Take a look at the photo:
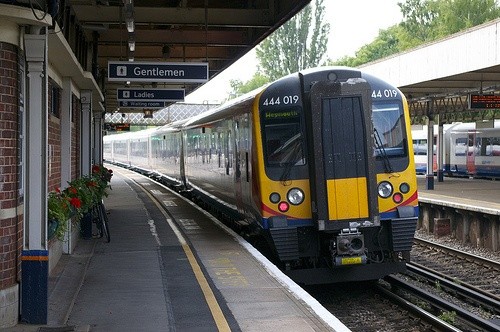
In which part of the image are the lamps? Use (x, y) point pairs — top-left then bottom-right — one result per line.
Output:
(125, 1), (136, 62)
(143, 110), (154, 118)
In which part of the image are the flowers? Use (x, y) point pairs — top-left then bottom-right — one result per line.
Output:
(48, 165), (113, 240)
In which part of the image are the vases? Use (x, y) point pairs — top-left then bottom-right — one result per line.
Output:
(48, 221), (58, 240)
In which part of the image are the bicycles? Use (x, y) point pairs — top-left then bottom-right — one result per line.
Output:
(82, 172), (112, 242)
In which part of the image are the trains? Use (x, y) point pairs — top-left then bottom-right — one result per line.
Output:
(409, 118), (500, 178)
(102, 66), (419, 286)
